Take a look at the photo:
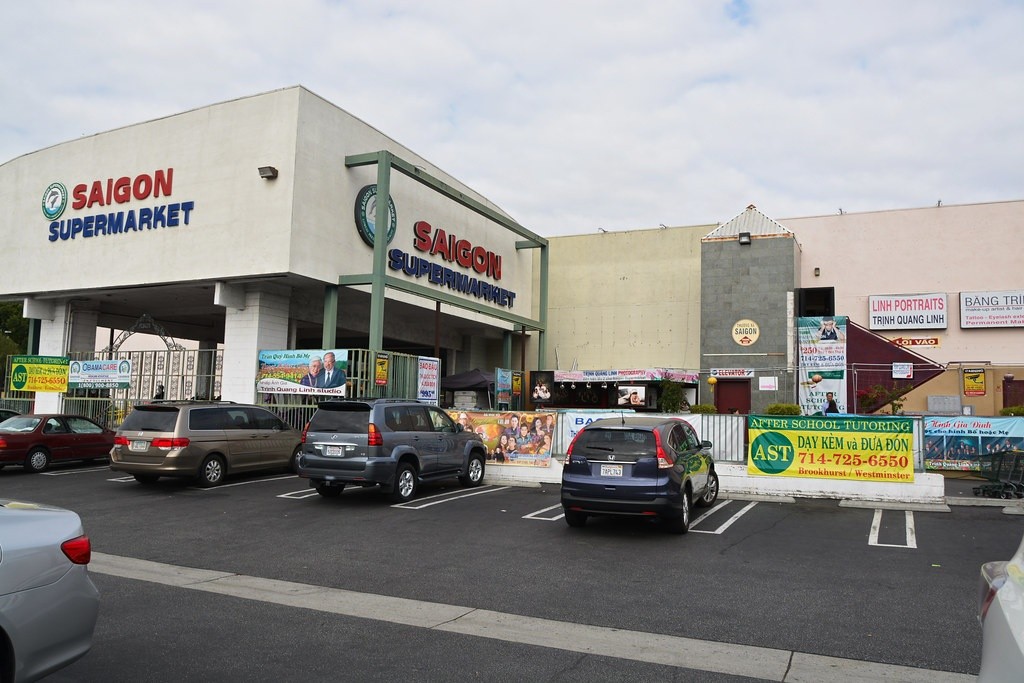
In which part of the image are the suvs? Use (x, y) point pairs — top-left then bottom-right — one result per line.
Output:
(299, 396), (488, 504)
(108, 398), (304, 488)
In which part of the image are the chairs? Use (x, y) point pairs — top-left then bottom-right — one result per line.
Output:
(234, 416), (246, 427)
(45, 423), (52, 431)
(408, 416), (418, 425)
(611, 434), (625, 442)
(60, 424), (69, 429)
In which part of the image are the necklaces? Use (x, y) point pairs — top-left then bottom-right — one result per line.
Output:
(307, 371), (318, 388)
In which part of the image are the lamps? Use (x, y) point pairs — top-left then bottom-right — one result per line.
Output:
(738, 232), (751, 245)
(601, 382), (607, 389)
(586, 382), (591, 389)
(659, 224), (666, 228)
(815, 268), (820, 276)
(599, 228), (607, 232)
(838, 209), (843, 215)
(560, 382), (565, 389)
(614, 382), (619, 387)
(257, 166), (277, 179)
(937, 201), (942, 206)
(571, 382), (576, 389)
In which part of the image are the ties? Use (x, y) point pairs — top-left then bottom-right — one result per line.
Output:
(326, 372), (331, 386)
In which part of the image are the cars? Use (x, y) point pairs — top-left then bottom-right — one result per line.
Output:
(561, 416), (719, 535)
(0, 497), (101, 683)
(0, 414), (117, 472)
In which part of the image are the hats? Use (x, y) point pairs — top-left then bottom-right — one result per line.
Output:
(630, 389), (638, 394)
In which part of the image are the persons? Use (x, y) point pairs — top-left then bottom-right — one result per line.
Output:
(152, 385), (165, 400)
(299, 352), (345, 391)
(627, 391), (642, 406)
(925, 438), (1020, 462)
(728, 408), (740, 414)
(816, 317), (845, 343)
(819, 392), (840, 415)
(457, 412), (555, 465)
(533, 383), (551, 399)
